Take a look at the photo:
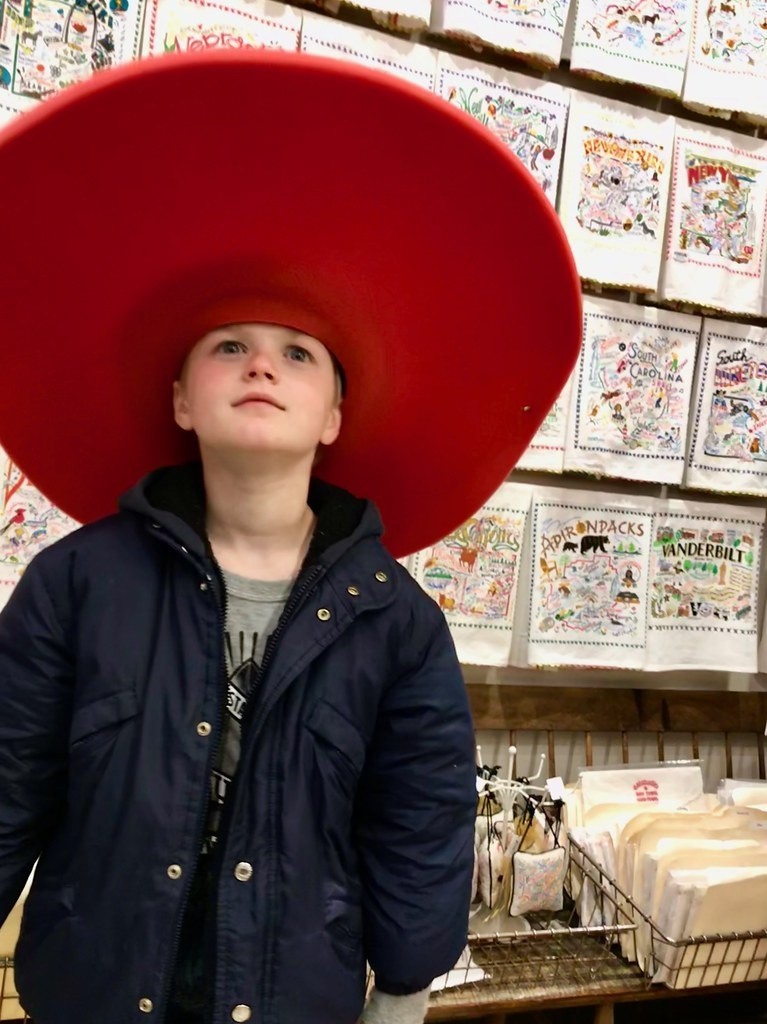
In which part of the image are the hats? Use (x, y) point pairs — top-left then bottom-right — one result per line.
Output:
(2, 48), (582, 558)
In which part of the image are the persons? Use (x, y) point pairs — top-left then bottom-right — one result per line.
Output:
(0, 48), (583, 1024)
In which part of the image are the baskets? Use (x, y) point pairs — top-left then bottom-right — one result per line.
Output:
(567, 828), (767, 989)
(365, 805), (650, 1009)
